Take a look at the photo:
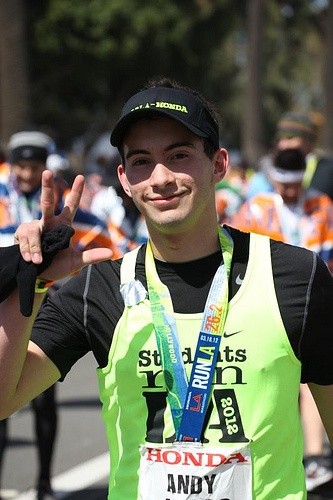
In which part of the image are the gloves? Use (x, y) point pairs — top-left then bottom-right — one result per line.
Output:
(0, 224), (76, 316)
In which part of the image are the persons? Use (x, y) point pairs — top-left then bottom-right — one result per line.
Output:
(231, 148), (333, 492)
(46, 108), (333, 262)
(1, 82), (333, 500)
(0, 130), (78, 500)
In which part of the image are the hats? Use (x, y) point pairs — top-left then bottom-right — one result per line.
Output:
(110, 87), (220, 152)
(276, 112), (318, 142)
(8, 130), (58, 166)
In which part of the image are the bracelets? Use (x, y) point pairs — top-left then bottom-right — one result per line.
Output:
(34, 278), (55, 295)
